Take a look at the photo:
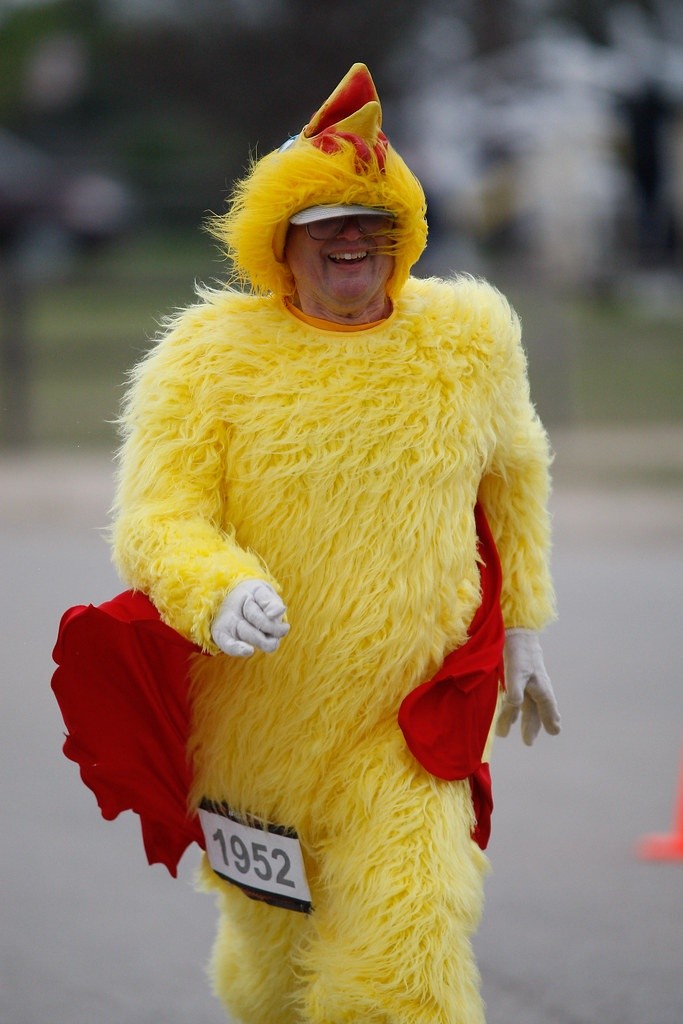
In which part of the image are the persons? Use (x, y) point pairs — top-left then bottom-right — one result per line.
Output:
(50, 56), (559, 1024)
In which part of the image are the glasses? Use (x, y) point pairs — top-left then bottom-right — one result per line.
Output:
(307, 214), (394, 238)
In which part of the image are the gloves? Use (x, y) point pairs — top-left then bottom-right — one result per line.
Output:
(213, 577), (289, 659)
(499, 629), (560, 748)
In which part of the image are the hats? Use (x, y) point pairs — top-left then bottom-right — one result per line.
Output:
(288, 201), (396, 224)
